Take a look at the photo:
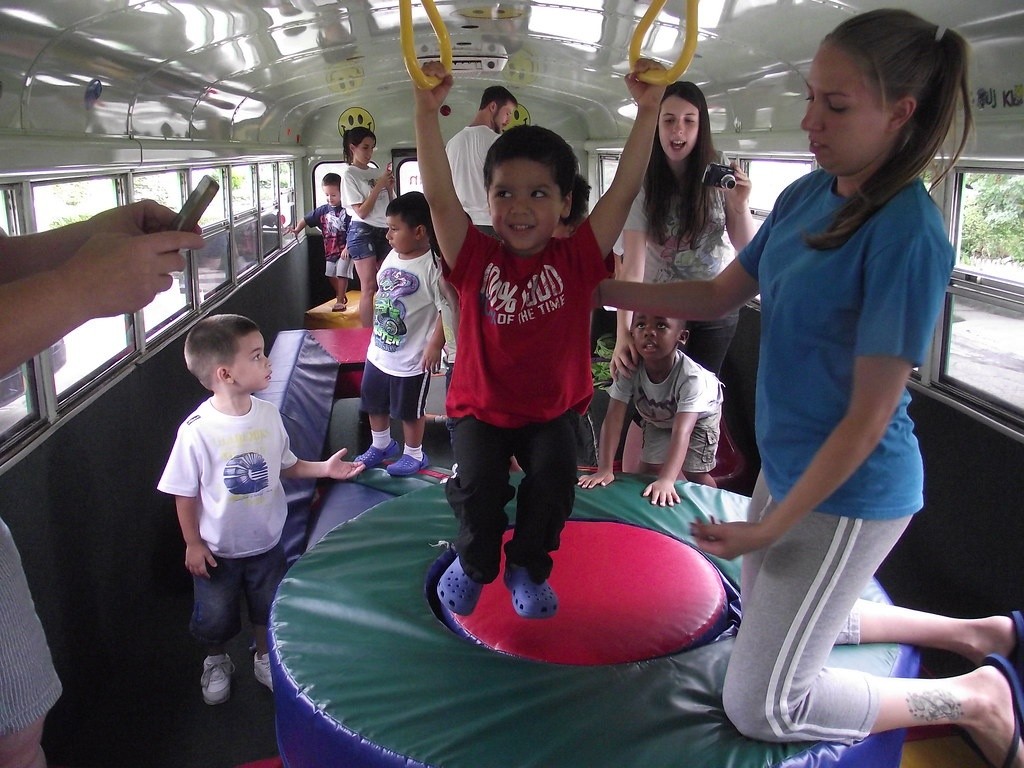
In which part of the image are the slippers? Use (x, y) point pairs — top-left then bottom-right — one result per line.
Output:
(961, 653), (1024, 768)
(1009, 610), (1024, 679)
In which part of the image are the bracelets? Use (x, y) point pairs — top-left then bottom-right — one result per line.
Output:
(598, 283), (604, 306)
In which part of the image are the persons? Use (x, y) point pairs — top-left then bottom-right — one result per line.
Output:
(552, 174), (591, 239)
(288, 173), (354, 312)
(595, 8), (1024, 768)
(157, 313), (366, 705)
(445, 85), (518, 240)
(0, 199), (207, 768)
(356, 190), (446, 476)
(610, 81), (756, 474)
(576, 310), (723, 507)
(341, 127), (397, 328)
(412, 58), (668, 619)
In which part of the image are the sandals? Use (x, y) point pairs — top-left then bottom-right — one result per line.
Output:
(436, 555), (481, 615)
(387, 452), (429, 476)
(354, 438), (400, 468)
(504, 561), (558, 619)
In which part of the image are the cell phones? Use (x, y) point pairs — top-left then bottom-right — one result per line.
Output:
(166, 174), (220, 254)
(387, 162), (392, 173)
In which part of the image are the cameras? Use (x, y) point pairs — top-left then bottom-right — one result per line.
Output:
(700, 162), (738, 191)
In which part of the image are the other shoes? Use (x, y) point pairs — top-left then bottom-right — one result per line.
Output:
(344, 297), (348, 304)
(332, 303), (346, 312)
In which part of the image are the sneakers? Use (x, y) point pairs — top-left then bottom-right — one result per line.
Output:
(253, 652), (274, 691)
(201, 653), (236, 705)
